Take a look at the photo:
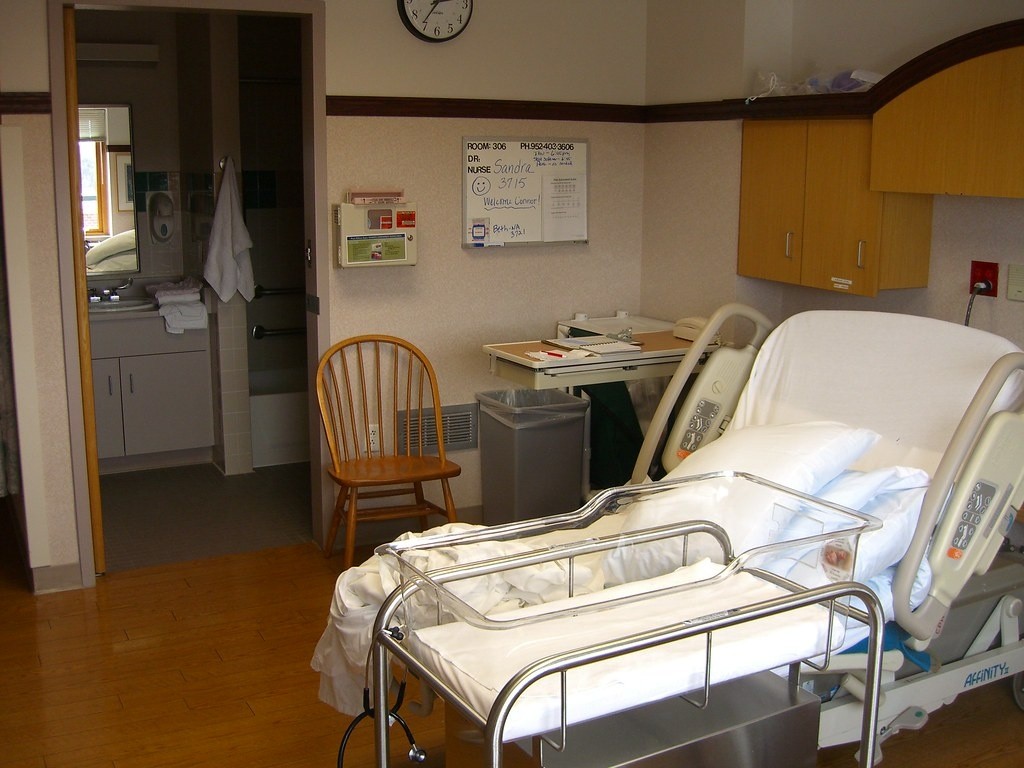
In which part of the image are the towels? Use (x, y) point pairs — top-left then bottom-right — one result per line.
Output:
(147, 276), (209, 335)
(202, 156), (258, 303)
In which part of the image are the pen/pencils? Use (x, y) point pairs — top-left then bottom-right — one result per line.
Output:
(540, 349), (566, 358)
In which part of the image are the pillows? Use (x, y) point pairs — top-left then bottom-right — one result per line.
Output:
(599, 420), (882, 588)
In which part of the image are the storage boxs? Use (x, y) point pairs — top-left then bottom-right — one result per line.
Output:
(331, 202), (418, 267)
(373, 466), (883, 739)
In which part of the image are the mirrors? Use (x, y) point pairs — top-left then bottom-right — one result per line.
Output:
(78, 103), (142, 275)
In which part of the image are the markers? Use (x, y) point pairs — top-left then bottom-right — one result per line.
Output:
(474, 243), (505, 247)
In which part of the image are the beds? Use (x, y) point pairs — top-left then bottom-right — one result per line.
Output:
(308, 306), (1024, 768)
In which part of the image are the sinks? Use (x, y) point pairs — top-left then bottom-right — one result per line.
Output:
(86, 297), (156, 312)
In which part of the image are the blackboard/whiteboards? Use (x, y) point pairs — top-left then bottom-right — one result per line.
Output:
(461, 137), (590, 249)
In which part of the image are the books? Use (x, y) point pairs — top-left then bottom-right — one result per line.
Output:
(543, 334), (641, 356)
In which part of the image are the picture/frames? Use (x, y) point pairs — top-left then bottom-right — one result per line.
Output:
(115, 153), (134, 213)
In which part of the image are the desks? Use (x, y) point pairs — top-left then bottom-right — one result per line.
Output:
(482, 330), (733, 497)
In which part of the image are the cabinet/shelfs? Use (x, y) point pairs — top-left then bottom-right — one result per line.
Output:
(738, 116), (931, 298)
(90, 315), (216, 476)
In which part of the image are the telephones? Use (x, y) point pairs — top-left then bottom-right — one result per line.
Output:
(672, 316), (724, 346)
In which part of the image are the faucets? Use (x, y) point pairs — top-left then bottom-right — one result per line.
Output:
(103, 276), (133, 302)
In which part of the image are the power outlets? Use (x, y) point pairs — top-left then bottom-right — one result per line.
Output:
(363, 424), (380, 452)
(967, 260), (999, 297)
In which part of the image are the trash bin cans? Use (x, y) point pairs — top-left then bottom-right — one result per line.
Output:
(474, 389), (588, 527)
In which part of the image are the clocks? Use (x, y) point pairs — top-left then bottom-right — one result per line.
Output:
(397, 0), (473, 43)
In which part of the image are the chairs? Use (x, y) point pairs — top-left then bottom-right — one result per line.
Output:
(315, 334), (463, 570)
